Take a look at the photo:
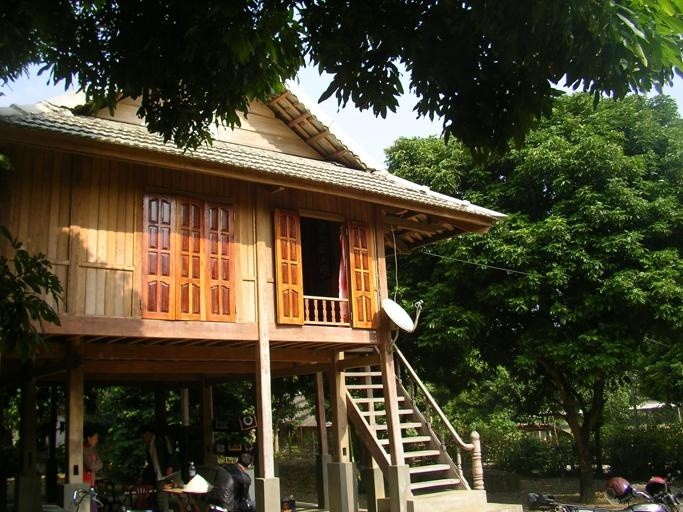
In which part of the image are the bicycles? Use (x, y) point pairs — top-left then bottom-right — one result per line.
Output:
(38, 487), (155, 512)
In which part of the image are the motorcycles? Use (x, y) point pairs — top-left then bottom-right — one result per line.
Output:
(522, 478), (682, 512)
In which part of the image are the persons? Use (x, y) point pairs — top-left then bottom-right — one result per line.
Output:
(135, 427), (175, 486)
(82, 426), (103, 512)
(207, 452), (252, 512)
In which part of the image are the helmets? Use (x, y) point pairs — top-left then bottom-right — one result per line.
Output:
(605, 477), (633, 506)
